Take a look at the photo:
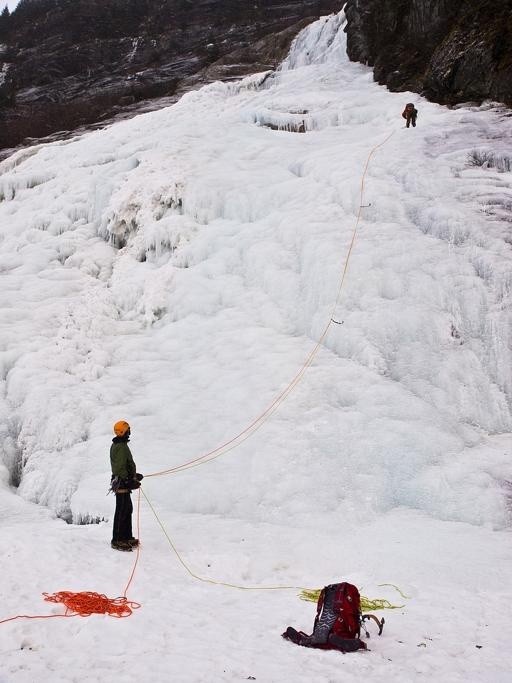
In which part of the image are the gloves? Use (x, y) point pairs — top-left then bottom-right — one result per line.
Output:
(134, 473), (143, 488)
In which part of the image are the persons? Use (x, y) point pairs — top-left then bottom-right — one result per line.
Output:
(111, 421), (139, 552)
(402, 103), (418, 128)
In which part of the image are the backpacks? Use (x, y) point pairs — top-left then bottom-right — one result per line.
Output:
(312, 582), (362, 653)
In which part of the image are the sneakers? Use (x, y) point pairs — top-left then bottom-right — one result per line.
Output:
(111, 537), (138, 550)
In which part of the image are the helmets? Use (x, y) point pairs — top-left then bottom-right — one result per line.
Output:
(114, 421), (129, 436)
